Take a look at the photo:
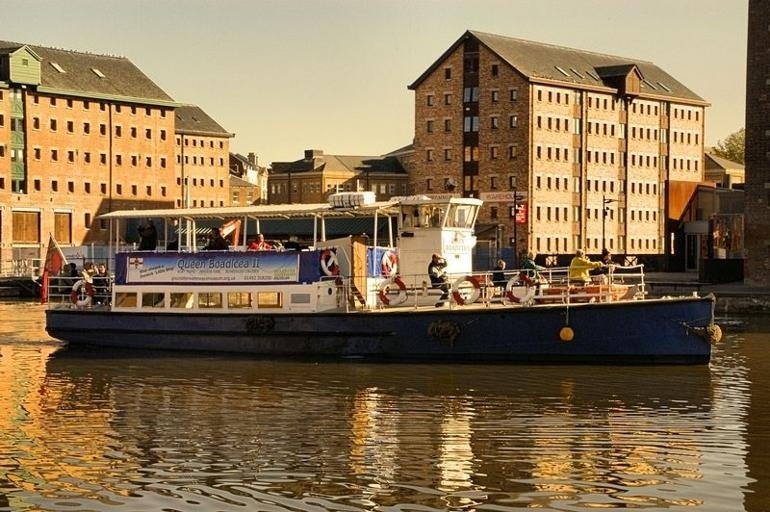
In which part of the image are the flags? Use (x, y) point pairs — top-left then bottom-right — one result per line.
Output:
(41, 237), (63, 305)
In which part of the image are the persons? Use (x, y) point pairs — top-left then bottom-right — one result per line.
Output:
(492, 259), (510, 296)
(201, 227), (229, 251)
(521, 249), (547, 297)
(137, 219), (158, 250)
(284, 236), (302, 250)
(569, 248), (605, 286)
(167, 233), (183, 251)
(595, 250), (622, 284)
(248, 233), (272, 251)
(271, 239), (286, 251)
(428, 254), (450, 307)
(57, 261), (113, 306)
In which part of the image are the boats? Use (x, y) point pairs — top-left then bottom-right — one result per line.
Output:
(46, 191), (716, 366)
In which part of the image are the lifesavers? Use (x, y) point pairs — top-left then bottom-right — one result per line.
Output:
(506, 274), (536, 303)
(380, 279), (406, 306)
(321, 250), (339, 276)
(382, 251), (397, 278)
(71, 280), (93, 306)
(452, 276), (480, 305)
